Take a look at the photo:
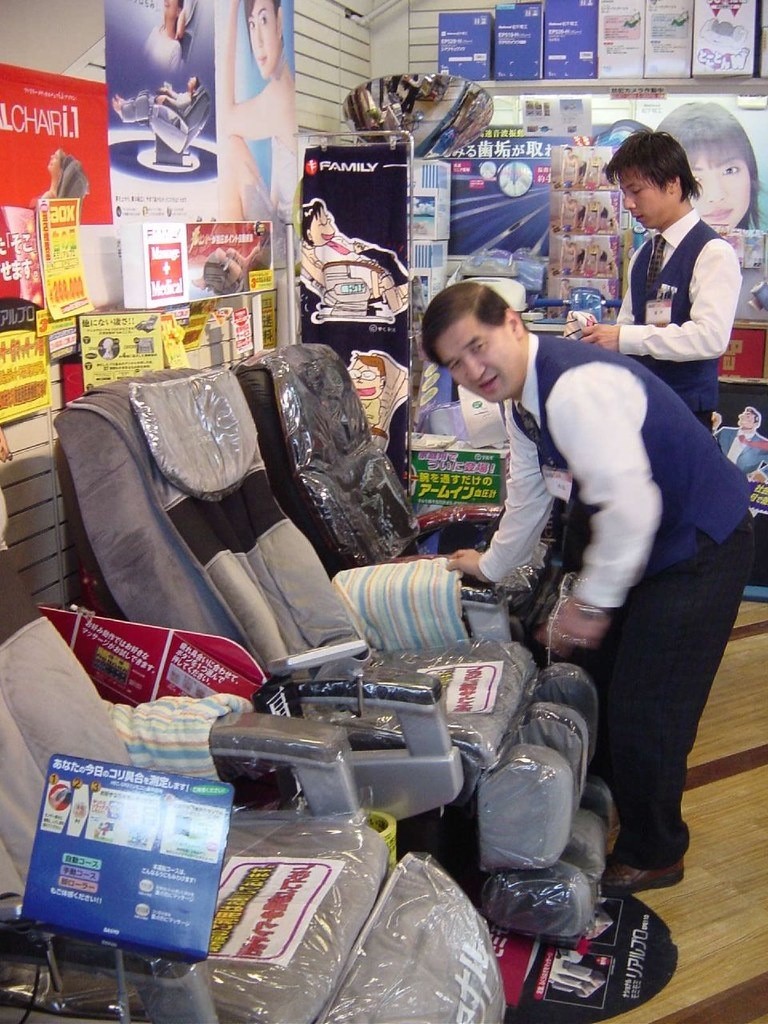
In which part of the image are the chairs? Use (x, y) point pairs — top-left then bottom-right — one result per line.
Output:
(51, 367), (618, 938)
(236, 344), (567, 632)
(0, 492), (505, 1024)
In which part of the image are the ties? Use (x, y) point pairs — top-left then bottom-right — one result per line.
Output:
(517, 400), (544, 451)
(645, 235), (666, 295)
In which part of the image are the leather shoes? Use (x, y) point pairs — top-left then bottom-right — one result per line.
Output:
(599, 853), (685, 900)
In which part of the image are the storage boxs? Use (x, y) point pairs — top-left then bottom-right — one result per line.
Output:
(494, 2), (543, 81)
(166, 297), (254, 369)
(437, 12), (494, 80)
(544, 0), (768, 80)
(121, 218), (274, 309)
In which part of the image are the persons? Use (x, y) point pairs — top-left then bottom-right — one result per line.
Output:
(421, 282), (756, 894)
(217, 0), (298, 266)
(41, 148), (67, 199)
(655, 101), (760, 229)
(112, 76), (200, 119)
(581, 130), (743, 431)
(145, 0), (183, 73)
